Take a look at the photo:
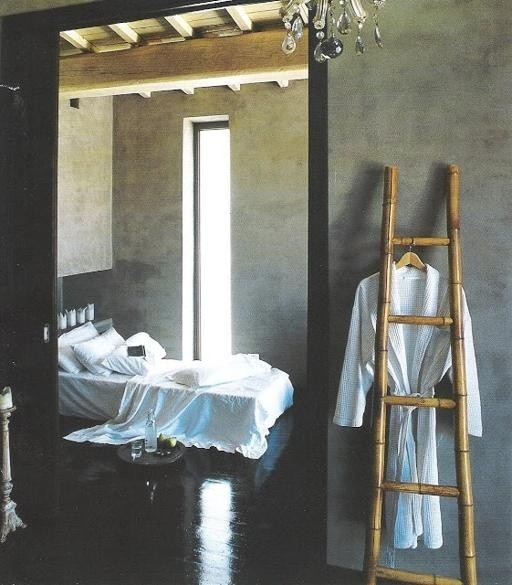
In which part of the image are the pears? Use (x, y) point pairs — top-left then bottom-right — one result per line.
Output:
(166, 438), (177, 447)
(160, 434), (168, 445)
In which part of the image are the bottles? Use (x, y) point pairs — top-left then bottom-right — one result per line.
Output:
(144, 409), (157, 453)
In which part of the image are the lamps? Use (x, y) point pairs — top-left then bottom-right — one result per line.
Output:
(279, 0), (388, 63)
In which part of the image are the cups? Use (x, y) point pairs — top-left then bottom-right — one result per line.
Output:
(130, 440), (142, 458)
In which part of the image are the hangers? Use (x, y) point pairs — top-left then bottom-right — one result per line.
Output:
(395, 238), (428, 273)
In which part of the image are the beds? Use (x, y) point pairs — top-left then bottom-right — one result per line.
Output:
(58, 360), (296, 460)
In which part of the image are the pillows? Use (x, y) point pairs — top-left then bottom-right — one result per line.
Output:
(161, 353), (271, 388)
(71, 327), (126, 376)
(58, 321), (100, 374)
(102, 332), (166, 376)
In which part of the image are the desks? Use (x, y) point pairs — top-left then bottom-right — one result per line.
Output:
(115, 437), (198, 500)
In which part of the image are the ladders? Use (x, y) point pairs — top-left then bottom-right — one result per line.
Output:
(365, 163), (478, 585)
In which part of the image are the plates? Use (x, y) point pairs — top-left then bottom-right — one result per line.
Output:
(117, 440), (186, 465)
